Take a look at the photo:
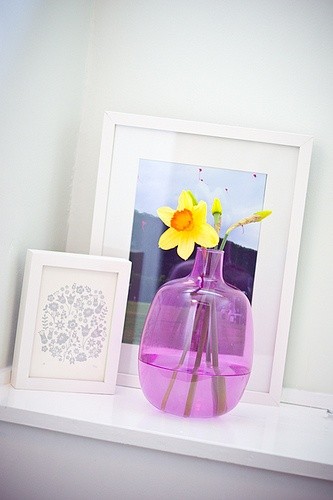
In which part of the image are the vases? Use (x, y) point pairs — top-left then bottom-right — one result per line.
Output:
(138, 247), (254, 419)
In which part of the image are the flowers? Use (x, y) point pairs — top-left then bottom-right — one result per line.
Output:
(156, 190), (273, 418)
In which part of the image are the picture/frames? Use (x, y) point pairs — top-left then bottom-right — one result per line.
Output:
(88, 111), (313, 408)
(9, 249), (132, 394)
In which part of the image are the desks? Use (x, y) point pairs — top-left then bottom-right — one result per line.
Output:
(0, 365), (333, 500)
